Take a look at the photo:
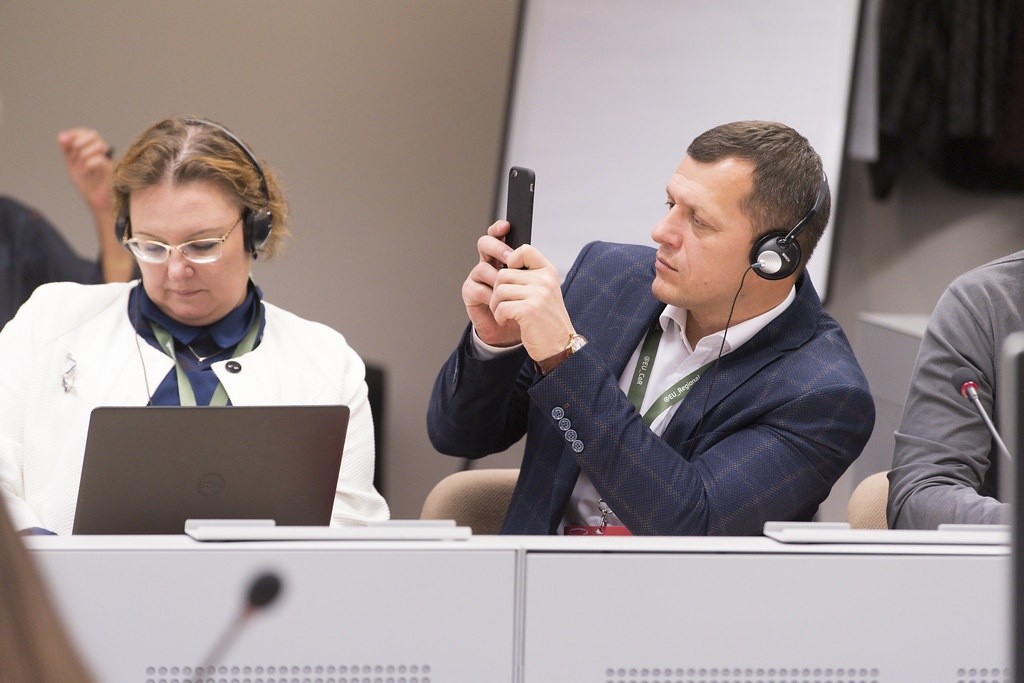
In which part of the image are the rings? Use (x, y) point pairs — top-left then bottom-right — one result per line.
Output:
(106, 146), (114, 157)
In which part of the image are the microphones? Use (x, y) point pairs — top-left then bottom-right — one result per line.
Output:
(951, 367), (1013, 465)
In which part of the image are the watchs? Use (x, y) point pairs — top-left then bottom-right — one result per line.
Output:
(537, 334), (589, 376)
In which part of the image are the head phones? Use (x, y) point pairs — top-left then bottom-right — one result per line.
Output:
(749, 172), (828, 281)
(113, 120), (273, 261)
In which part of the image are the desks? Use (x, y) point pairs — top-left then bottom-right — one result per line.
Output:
(854, 311), (938, 497)
(14, 534), (1013, 683)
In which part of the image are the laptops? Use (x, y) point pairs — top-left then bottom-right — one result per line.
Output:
(71, 405), (350, 534)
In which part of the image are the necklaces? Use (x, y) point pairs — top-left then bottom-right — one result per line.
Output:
(187, 345), (223, 364)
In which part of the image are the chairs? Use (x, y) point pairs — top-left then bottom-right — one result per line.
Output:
(849, 471), (889, 527)
(419, 464), (518, 528)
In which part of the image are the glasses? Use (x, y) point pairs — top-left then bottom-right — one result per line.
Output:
(120, 208), (246, 265)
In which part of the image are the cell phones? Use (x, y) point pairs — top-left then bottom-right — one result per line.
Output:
(503, 167), (536, 270)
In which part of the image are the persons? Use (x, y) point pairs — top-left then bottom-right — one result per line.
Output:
(0, 119), (390, 535)
(886, 250), (1024, 531)
(0, 128), (142, 333)
(426, 120), (875, 538)
